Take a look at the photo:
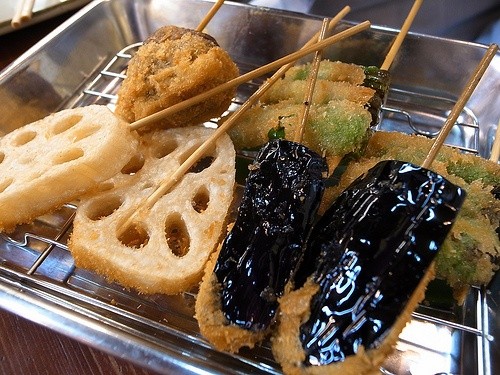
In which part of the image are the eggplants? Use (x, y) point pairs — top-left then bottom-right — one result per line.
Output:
(267, 160), (466, 375)
(197, 138), (329, 353)
